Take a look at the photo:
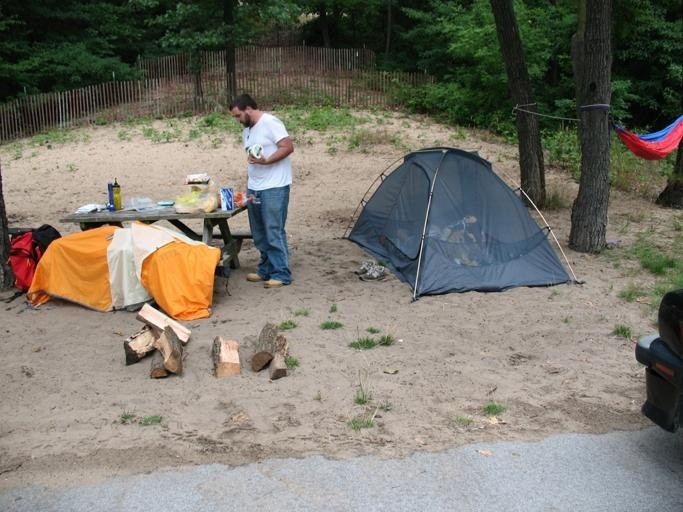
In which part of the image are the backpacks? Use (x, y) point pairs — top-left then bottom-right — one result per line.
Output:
(10, 224), (62, 290)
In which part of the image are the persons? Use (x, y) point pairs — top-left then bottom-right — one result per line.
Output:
(230, 93), (294, 287)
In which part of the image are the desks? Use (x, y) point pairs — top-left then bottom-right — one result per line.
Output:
(59, 203), (253, 278)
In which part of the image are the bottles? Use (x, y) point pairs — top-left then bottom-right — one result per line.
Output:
(233, 195), (253, 209)
(111, 178), (121, 211)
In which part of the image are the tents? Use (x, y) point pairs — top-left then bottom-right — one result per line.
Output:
(348, 147), (572, 295)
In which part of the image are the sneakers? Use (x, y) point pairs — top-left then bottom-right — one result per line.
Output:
(356, 260), (384, 282)
(264, 279), (283, 288)
(247, 273), (265, 282)
